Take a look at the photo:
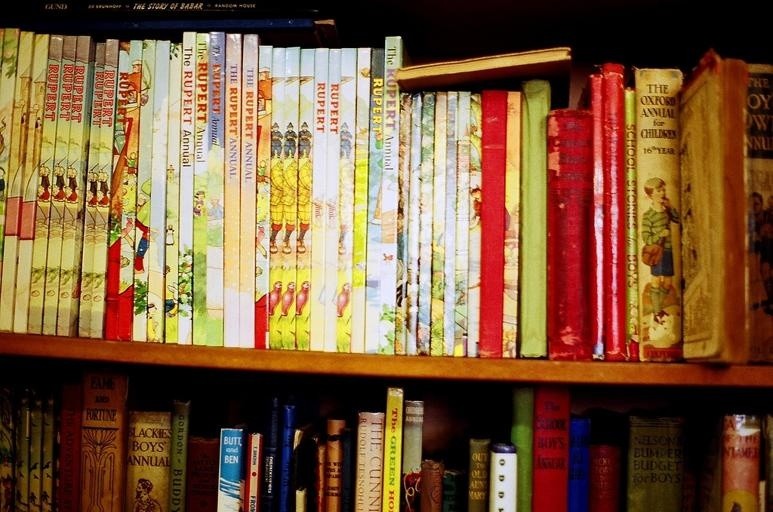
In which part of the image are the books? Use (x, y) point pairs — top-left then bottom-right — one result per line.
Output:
(2, 29), (772, 511)
